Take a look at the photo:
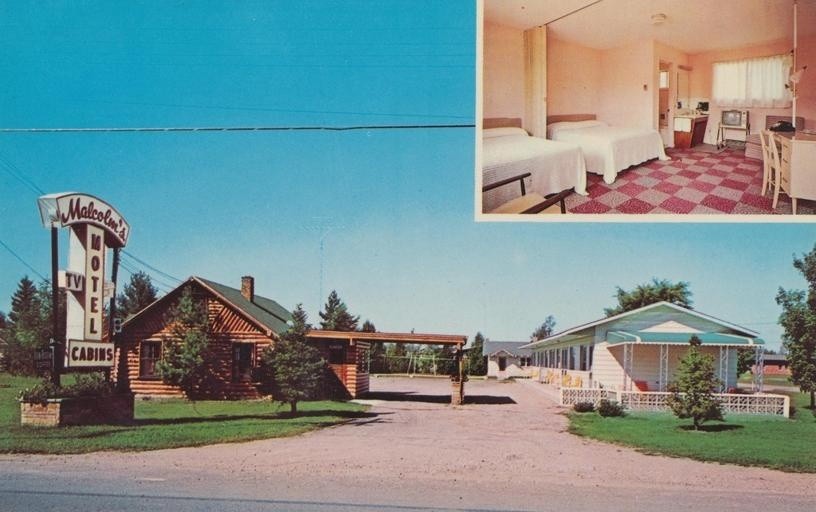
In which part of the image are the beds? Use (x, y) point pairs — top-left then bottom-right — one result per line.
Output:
(546, 115), (672, 185)
(482, 117), (582, 214)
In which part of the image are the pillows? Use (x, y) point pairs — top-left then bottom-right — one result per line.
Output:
(482, 126), (528, 139)
(548, 120), (609, 131)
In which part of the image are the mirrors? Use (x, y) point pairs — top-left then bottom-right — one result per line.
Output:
(676, 72), (691, 108)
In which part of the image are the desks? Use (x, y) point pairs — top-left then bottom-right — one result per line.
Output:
(674, 113), (708, 148)
(761, 126), (815, 215)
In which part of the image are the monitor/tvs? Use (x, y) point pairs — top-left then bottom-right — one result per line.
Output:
(722, 111), (749, 128)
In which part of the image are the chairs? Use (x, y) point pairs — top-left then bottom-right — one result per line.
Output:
(482, 172), (574, 214)
(752, 130), (783, 208)
(744, 116), (804, 163)
